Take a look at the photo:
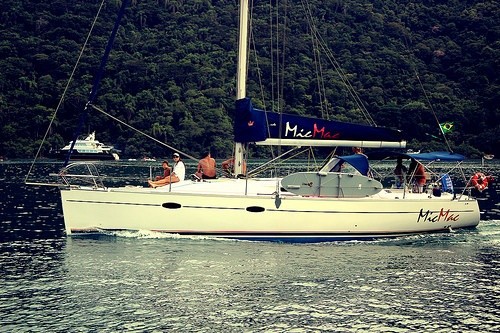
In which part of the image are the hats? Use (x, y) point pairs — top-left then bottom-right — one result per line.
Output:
(173, 152), (180, 156)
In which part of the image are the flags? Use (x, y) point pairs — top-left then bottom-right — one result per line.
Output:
(439, 121), (456, 134)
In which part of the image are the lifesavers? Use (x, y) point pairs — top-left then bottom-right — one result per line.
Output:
(473, 172), (488, 191)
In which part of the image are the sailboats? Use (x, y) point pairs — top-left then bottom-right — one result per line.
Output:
(24, 0), (480, 236)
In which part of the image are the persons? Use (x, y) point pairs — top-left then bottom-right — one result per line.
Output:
(148, 153), (185, 188)
(195, 151), (216, 179)
(148, 161), (171, 188)
(406, 158), (426, 193)
(354, 148), (368, 175)
(394, 158), (407, 189)
(222, 153), (246, 178)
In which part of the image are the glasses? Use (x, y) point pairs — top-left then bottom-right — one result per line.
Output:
(173, 156), (179, 158)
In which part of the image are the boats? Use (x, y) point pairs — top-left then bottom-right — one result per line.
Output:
(60, 130), (120, 161)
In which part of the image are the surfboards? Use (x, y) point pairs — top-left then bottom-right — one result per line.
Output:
(281, 172), (383, 198)
